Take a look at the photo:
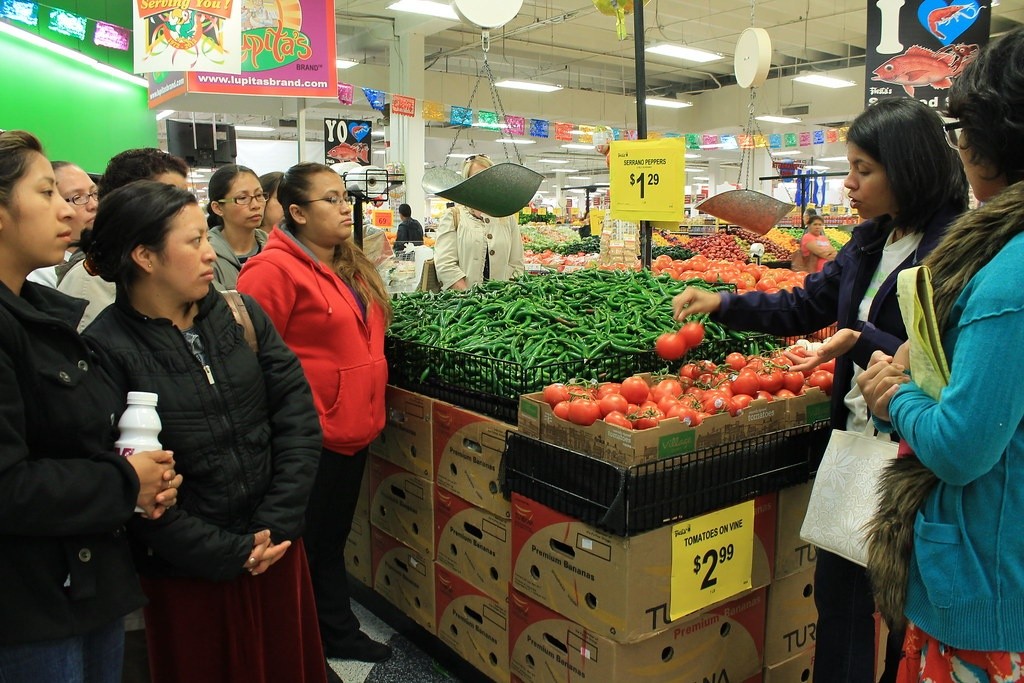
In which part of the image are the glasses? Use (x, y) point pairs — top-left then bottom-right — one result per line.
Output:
(297, 195), (357, 207)
(65, 189), (99, 205)
(218, 192), (270, 205)
(942, 119), (967, 151)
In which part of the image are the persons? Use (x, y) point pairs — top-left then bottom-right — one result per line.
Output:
(56, 147), (188, 335)
(803, 208), (825, 235)
(236, 161), (393, 683)
(579, 212), (591, 241)
(206, 164), (270, 292)
(26, 161), (98, 289)
(82, 179), (328, 683)
(856, 31), (1024, 683)
(800, 216), (838, 273)
(393, 204), (423, 251)
(258, 172), (285, 235)
(0, 129), (183, 683)
(670, 97), (969, 683)
(434, 152), (525, 292)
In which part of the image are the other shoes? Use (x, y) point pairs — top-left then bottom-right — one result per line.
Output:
(327, 665), (344, 683)
(324, 629), (391, 661)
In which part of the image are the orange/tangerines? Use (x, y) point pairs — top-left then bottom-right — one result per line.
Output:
(423, 237), (435, 246)
(386, 232), (397, 246)
(765, 229), (804, 252)
(824, 227), (851, 251)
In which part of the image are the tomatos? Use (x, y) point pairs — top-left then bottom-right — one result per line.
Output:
(596, 254), (812, 293)
(542, 321), (836, 434)
(524, 250), (596, 270)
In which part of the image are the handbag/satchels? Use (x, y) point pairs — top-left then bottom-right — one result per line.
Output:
(417, 209), (461, 291)
(800, 416), (900, 570)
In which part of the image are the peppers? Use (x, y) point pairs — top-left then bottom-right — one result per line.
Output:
(380, 263), (781, 402)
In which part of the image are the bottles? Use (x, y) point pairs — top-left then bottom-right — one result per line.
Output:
(115, 391), (163, 513)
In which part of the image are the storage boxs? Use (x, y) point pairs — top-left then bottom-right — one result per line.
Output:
(345, 385), (890, 683)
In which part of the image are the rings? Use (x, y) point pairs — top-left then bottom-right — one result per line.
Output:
(168, 480), (171, 487)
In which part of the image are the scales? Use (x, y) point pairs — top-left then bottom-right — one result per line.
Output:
(420, 0), (545, 218)
(694, 27), (797, 236)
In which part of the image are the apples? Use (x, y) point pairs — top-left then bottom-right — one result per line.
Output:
(682, 227), (794, 263)
(520, 226), (581, 254)
